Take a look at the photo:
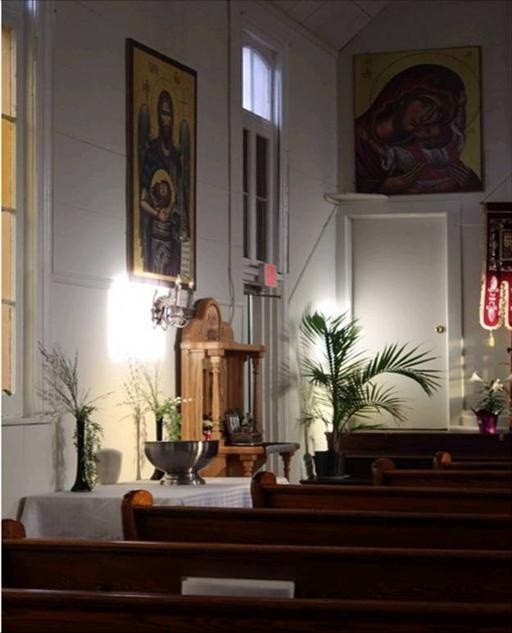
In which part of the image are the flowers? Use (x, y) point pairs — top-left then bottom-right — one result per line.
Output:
(468, 370), (508, 410)
(34, 340), (115, 493)
(139, 363), (187, 440)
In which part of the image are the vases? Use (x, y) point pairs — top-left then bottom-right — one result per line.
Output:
(149, 418), (165, 480)
(70, 420), (91, 492)
(476, 412), (500, 436)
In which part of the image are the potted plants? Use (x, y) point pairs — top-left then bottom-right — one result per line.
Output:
(287, 306), (444, 477)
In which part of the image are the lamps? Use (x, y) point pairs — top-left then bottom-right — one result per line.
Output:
(151, 274), (196, 331)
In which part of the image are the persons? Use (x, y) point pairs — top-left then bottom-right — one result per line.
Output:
(138, 86), (191, 278)
(355, 63), (481, 190)
(380, 88), (469, 174)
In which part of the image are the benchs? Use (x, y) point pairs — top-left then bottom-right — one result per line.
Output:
(1, 451), (512, 633)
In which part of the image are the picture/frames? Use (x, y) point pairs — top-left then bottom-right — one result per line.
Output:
(225, 413), (240, 435)
(125, 38), (198, 290)
